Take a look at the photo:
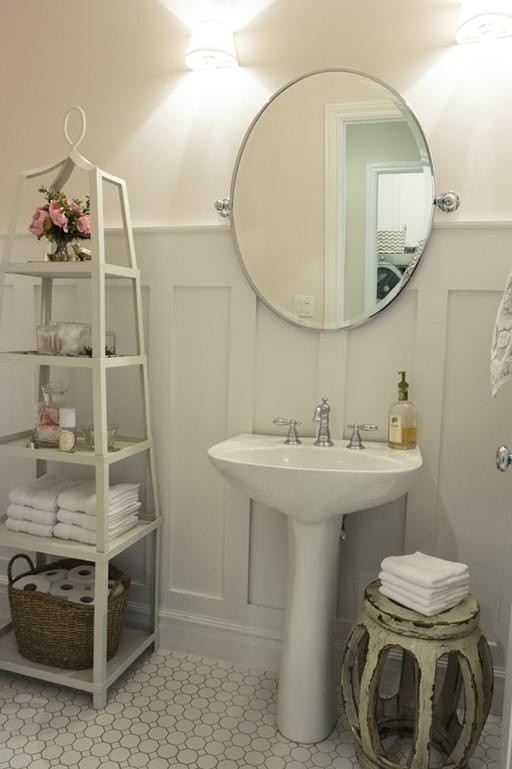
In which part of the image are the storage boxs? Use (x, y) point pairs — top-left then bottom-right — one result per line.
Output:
(373, 222), (409, 255)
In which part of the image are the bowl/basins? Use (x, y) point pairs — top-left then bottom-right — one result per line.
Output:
(83, 421), (121, 449)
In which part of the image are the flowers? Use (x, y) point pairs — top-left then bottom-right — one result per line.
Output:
(30, 183), (92, 246)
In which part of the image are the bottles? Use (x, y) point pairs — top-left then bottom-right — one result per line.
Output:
(30, 379), (70, 450)
(36, 321), (61, 356)
(59, 407), (77, 450)
(103, 333), (117, 354)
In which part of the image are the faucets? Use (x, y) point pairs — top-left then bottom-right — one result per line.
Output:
(313, 397), (333, 447)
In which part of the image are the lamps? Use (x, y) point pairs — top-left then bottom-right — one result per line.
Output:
(181, 18), (236, 77)
(451, 1), (512, 50)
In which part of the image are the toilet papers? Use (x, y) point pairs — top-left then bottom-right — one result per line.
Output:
(11, 565), (125, 606)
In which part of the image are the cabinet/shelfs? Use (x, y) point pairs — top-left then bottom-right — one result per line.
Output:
(0, 104), (167, 705)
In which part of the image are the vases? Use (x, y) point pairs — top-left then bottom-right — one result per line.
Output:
(51, 238), (69, 261)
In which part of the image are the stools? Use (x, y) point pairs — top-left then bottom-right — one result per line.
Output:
(338, 580), (497, 769)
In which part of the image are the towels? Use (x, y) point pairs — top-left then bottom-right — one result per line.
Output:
(490, 270), (511, 401)
(375, 549), (472, 619)
(4, 469), (143, 545)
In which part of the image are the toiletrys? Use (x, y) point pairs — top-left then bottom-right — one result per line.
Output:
(389, 371), (417, 451)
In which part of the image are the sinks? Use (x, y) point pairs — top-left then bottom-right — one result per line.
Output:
(208, 432), (423, 521)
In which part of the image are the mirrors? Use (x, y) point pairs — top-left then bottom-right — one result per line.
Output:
(215, 65), (462, 337)
